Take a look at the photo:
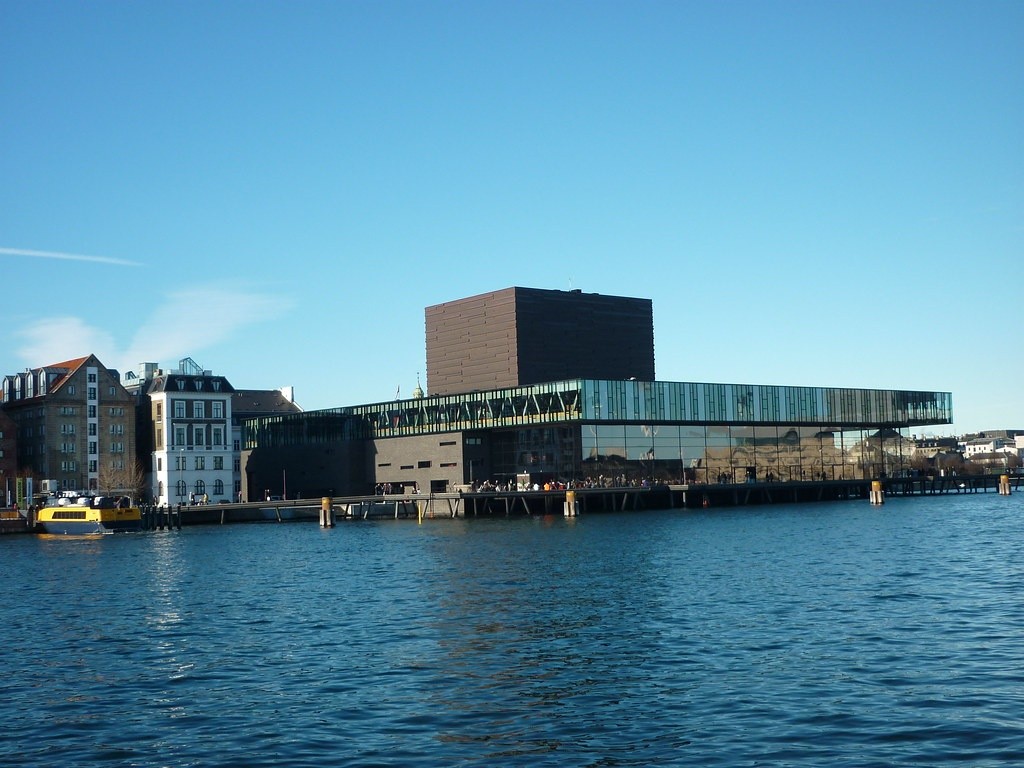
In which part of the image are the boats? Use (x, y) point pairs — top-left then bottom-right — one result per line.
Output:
(37, 495), (142, 535)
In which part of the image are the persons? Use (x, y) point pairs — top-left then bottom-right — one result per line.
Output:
(547, 473), (690, 487)
(374, 482), (393, 495)
(189, 492), (202, 506)
(717, 472), (731, 484)
(238, 491), (242, 503)
(821, 472), (827, 481)
(906, 468), (913, 478)
(945, 465), (957, 477)
(879, 470), (886, 478)
(203, 493), (208, 505)
(473, 479), (500, 492)
(766, 472), (774, 483)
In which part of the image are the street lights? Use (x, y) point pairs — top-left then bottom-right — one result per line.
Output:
(180, 448), (185, 507)
